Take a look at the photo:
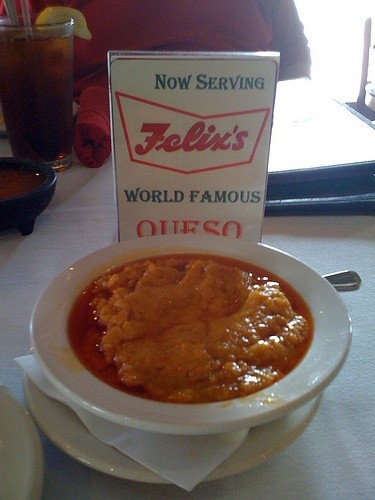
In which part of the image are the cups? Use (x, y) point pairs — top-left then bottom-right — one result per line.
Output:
(0, 13), (75, 171)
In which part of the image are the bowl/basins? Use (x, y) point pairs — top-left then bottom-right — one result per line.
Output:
(0, 156), (57, 236)
(30, 232), (353, 435)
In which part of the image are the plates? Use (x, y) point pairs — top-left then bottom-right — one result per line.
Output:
(0, 384), (45, 500)
(23, 348), (324, 484)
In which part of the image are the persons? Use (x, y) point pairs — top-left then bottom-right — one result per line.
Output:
(0, 0), (312, 106)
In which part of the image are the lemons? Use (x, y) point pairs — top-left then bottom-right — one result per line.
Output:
(34, 7), (92, 41)
